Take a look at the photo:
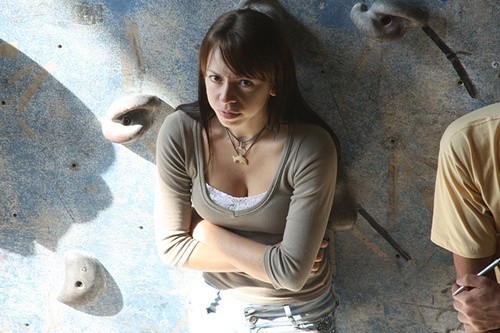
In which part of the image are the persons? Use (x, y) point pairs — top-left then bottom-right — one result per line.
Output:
(431, 102), (500, 333)
(156, 8), (340, 333)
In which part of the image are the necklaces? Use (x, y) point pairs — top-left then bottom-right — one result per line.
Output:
(224, 115), (270, 163)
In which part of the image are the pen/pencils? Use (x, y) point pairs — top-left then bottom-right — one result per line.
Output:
(453, 258), (500, 297)
